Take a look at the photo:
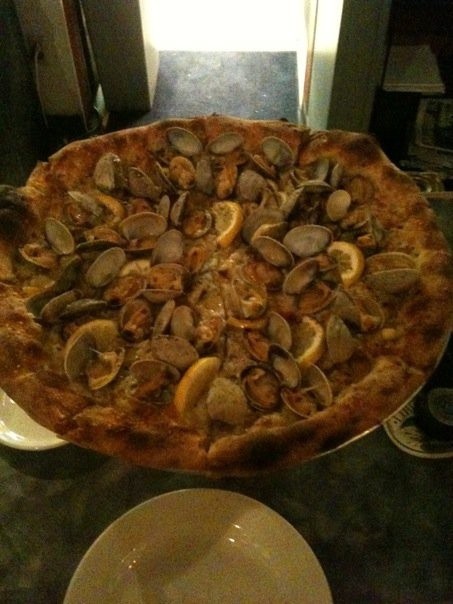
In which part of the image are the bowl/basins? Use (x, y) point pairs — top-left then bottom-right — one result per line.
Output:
(384, 353), (452, 459)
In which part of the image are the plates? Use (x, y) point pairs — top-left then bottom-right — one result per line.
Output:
(0, 391), (70, 452)
(62, 487), (334, 603)
